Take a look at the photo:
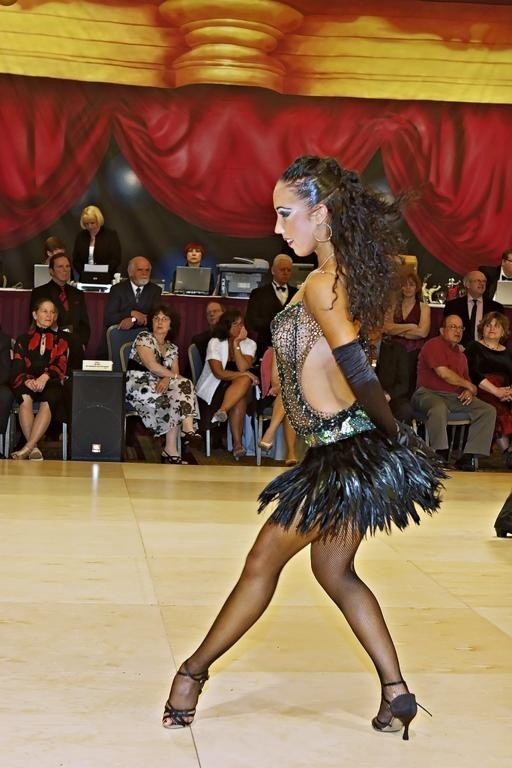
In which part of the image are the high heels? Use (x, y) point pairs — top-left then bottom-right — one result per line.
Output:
(211, 409), (227, 427)
(286, 459), (297, 467)
(233, 447), (247, 461)
(258, 441), (273, 455)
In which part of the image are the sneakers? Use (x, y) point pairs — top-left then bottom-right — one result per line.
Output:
(455, 454), (473, 471)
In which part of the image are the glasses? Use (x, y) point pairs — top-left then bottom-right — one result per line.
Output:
(232, 321), (244, 327)
(447, 324), (465, 331)
(470, 279), (487, 283)
(153, 317), (169, 321)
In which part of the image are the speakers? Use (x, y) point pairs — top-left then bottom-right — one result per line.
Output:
(70, 371), (124, 462)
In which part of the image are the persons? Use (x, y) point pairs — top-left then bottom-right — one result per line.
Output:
(162, 153), (453, 740)
(70, 202), (122, 285)
(493, 491), (511, 538)
(0, 232), (512, 471)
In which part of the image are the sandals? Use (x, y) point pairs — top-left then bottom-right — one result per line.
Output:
(372, 680), (418, 741)
(162, 660), (209, 729)
(180, 430), (202, 445)
(29, 447), (43, 461)
(161, 449), (189, 465)
(10, 447), (31, 459)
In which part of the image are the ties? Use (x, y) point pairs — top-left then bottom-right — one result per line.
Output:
(470, 299), (478, 341)
(136, 288), (141, 304)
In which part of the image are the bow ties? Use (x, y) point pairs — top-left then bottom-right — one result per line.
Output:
(276, 286), (286, 292)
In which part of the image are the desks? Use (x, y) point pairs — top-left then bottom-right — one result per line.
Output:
(1, 287), (512, 379)
